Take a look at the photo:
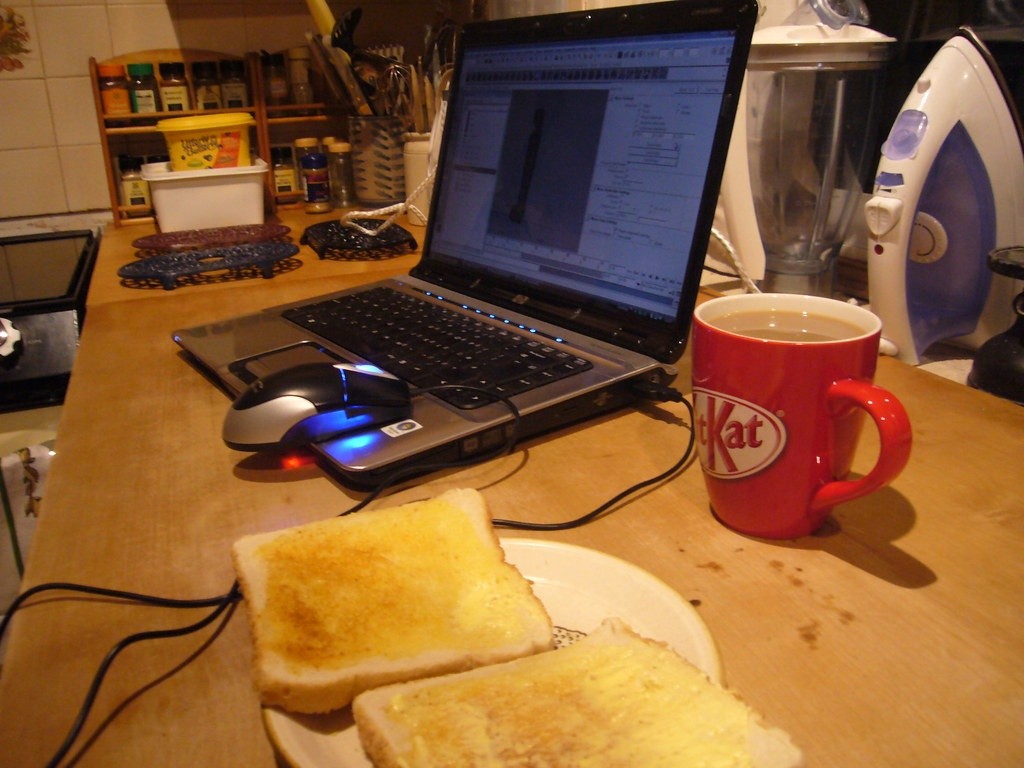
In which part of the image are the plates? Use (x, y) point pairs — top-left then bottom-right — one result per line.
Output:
(260, 539), (720, 768)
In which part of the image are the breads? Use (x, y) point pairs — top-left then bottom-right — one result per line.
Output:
(229, 487), (802, 767)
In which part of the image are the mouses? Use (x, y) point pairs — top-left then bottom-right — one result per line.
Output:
(220, 362), (410, 452)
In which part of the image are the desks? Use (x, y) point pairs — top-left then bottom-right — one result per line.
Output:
(0, 201), (1024, 768)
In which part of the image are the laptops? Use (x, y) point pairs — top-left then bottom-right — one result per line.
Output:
(171, 1), (760, 492)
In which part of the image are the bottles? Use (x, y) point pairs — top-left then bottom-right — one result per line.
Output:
(99, 46), (352, 215)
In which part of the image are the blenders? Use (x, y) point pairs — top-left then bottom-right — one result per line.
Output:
(744, 1), (898, 297)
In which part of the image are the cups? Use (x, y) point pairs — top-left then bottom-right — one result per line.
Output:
(403, 131), (430, 226)
(691, 291), (912, 537)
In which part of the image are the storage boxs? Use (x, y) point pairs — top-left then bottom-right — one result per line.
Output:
(136, 109), (266, 226)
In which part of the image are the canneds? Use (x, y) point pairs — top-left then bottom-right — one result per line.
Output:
(118, 155), (166, 215)
(270, 137), (356, 213)
(97, 58), (251, 114)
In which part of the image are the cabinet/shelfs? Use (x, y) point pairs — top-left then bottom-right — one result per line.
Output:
(262, 51), (345, 202)
(90, 48), (267, 227)
